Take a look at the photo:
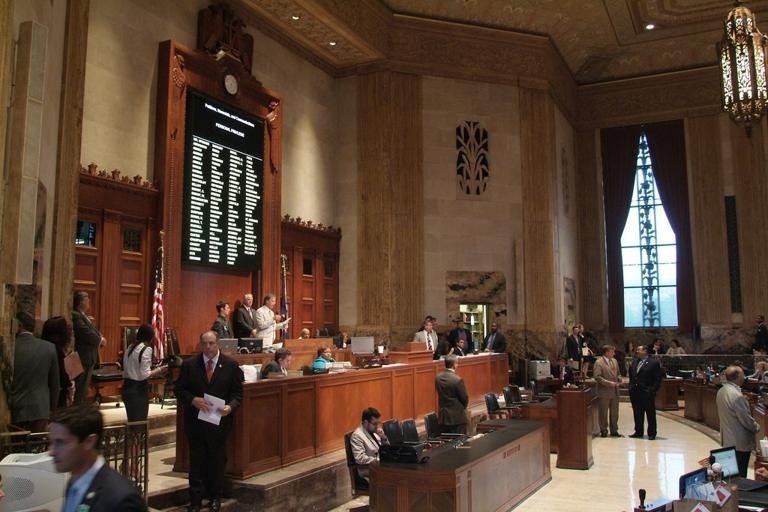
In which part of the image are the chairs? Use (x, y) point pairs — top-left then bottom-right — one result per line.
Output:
(124, 324), (169, 410)
(503, 385), (539, 410)
(425, 412), (467, 444)
(531, 379), (554, 401)
(486, 392), (521, 421)
(401, 421), (443, 448)
(382, 418), (425, 458)
(92, 359), (126, 408)
(174, 331), (204, 360)
(344, 429), (369, 497)
(161, 329), (195, 410)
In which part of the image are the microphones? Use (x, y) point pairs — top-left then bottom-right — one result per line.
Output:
(638, 489), (647, 510)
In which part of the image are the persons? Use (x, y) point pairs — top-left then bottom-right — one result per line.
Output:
(7, 311), (60, 439)
(451, 339), (466, 358)
(414, 314), (437, 354)
(262, 347), (291, 377)
(717, 365), (759, 479)
(551, 358), (573, 387)
(448, 319), (472, 352)
(175, 331), (244, 510)
(212, 302), (233, 338)
(649, 338), (664, 356)
(481, 323), (507, 355)
(120, 324), (163, 422)
(257, 296), (282, 349)
(434, 354), (470, 434)
(351, 407), (390, 485)
(667, 340), (685, 356)
(628, 345), (660, 440)
(566, 323), (596, 378)
(235, 292), (260, 336)
(747, 360), (768, 381)
(310, 347), (337, 371)
(625, 341), (633, 356)
(49, 407), (146, 512)
(593, 346), (624, 437)
(298, 328), (308, 341)
(72, 290), (106, 409)
(336, 334), (351, 347)
(40, 314), (76, 412)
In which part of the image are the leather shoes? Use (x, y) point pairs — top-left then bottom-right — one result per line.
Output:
(629, 433), (643, 438)
(649, 434), (655, 440)
(610, 433), (621, 437)
(188, 502), (202, 512)
(210, 499), (221, 512)
(601, 432), (607, 437)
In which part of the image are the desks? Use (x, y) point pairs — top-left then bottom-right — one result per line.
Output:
(683, 378), (708, 421)
(521, 384), (607, 452)
(701, 384), (723, 432)
(369, 420), (552, 511)
(655, 373), (680, 410)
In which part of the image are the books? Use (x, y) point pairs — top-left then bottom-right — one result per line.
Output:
(216, 337), (238, 355)
(679, 468), (720, 505)
(242, 338), (264, 354)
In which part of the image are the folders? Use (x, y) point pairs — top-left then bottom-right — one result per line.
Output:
(737, 489), (768, 508)
(63, 351), (84, 380)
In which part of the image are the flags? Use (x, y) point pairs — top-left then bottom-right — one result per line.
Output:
(281, 266), (290, 340)
(152, 246), (168, 391)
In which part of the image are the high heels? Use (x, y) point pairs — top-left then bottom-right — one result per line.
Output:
(129, 468), (148, 481)
(119, 464), (128, 478)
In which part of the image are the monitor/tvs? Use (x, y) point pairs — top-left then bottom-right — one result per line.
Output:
(242, 337), (263, 355)
(679, 467), (708, 501)
(218, 338), (239, 355)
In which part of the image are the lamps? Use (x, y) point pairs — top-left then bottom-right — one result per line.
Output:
(721, 1), (768, 133)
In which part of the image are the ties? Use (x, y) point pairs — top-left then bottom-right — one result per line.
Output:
(206, 360), (213, 382)
(248, 309), (252, 318)
(461, 332), (464, 340)
(637, 361), (643, 375)
(369, 430), (381, 447)
(269, 310), (271, 318)
(609, 359), (615, 372)
(489, 334), (495, 350)
(63, 487), (78, 512)
(428, 333), (432, 350)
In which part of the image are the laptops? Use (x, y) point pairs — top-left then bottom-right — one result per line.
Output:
(710, 445), (766, 492)
